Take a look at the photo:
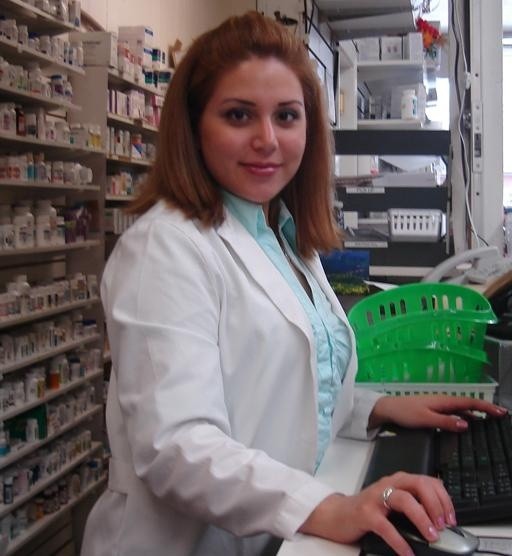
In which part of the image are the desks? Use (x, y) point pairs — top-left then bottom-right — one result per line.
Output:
(266, 408), (512, 556)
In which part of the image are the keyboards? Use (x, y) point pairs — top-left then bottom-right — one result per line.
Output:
(357, 412), (512, 526)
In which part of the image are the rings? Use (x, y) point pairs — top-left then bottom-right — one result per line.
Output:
(381, 484), (394, 509)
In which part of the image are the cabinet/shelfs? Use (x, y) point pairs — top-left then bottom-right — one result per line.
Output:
(350, 53), (428, 231)
(300, 0), (463, 297)
(67, 31), (180, 490)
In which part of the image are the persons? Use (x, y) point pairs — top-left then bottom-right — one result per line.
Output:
(76, 11), (509, 556)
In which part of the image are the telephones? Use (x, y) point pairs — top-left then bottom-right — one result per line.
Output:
(420, 246), (511, 295)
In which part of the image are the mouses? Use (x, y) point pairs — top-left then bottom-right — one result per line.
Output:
(362, 518), (480, 555)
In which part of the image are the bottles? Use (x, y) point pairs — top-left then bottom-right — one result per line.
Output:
(5, 188), (91, 247)
(3, 14), (84, 72)
(1, 141), (95, 185)
(1, 243), (105, 553)
(399, 88), (418, 121)
(24, 0), (83, 28)
(3, 52), (76, 104)
(2, 96), (104, 149)
